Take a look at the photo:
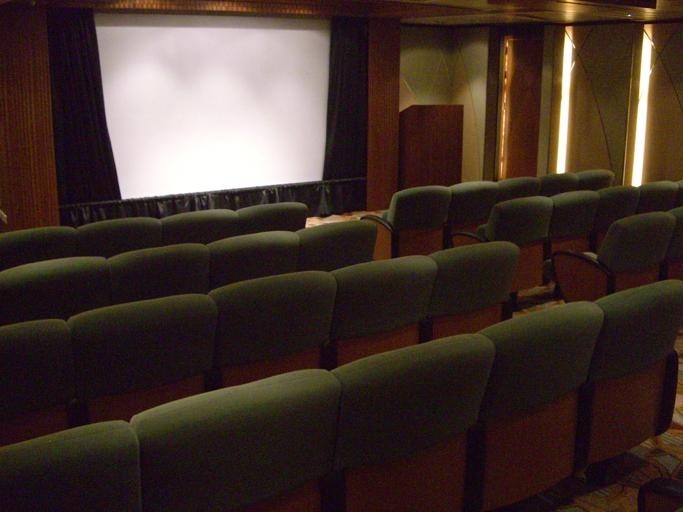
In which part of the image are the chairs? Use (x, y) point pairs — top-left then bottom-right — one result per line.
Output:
(1, 225), (78, 268)
(445, 170), (682, 282)
(299, 218), (379, 270)
(1, 255), (108, 324)
(322, 332), (497, 509)
(543, 211), (675, 310)
(107, 244), (210, 305)
(162, 210), (238, 245)
(430, 240), (521, 342)
(1, 420), (143, 512)
(572, 278), (682, 473)
(236, 201), (311, 237)
(68, 294), (218, 423)
(208, 231), (302, 288)
(360, 185), (449, 260)
(132, 370), (340, 510)
(75, 217), (163, 259)
(211, 271), (336, 390)
(463, 301), (604, 511)
(2, 319), (73, 441)
(451, 194), (552, 318)
(328, 253), (438, 368)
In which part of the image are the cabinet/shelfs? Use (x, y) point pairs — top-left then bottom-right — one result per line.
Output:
(398, 103), (463, 187)
